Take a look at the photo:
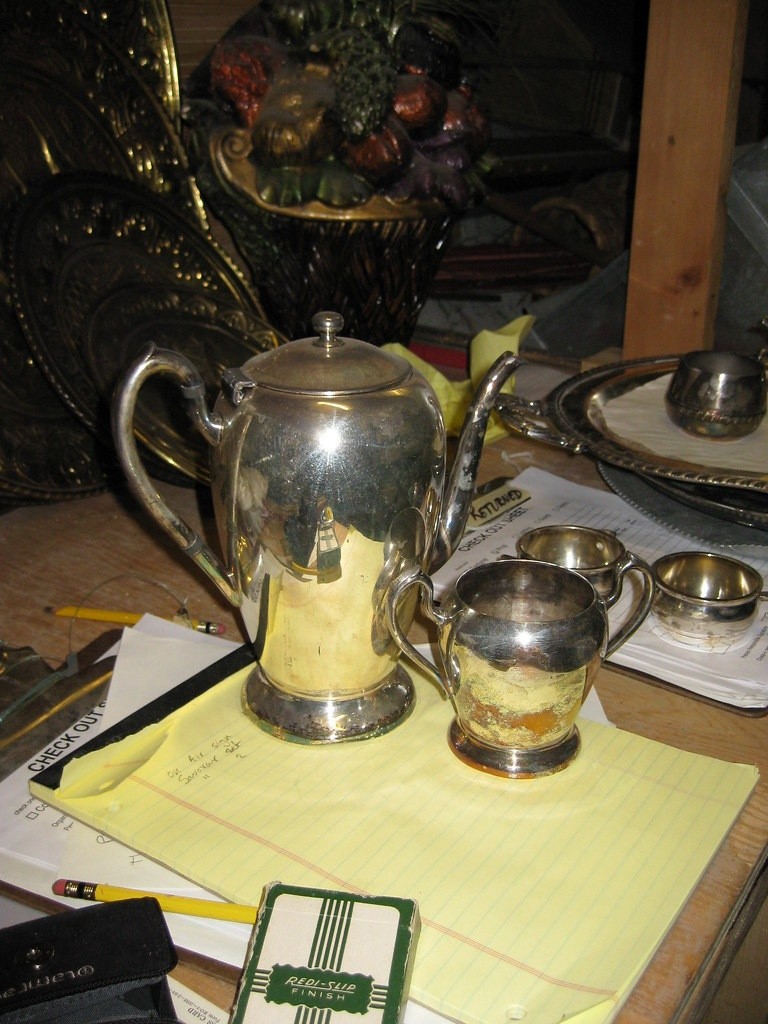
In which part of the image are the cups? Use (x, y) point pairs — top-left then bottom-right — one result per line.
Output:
(382, 560), (655, 781)
(502, 525), (626, 595)
(650, 552), (768, 649)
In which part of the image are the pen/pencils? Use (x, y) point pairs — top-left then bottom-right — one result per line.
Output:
(40, 603), (227, 634)
(52, 878), (259, 923)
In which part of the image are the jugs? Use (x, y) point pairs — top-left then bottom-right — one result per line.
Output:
(110, 312), (530, 745)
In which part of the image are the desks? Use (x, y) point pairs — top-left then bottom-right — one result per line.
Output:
(0, 324), (768, 1024)
